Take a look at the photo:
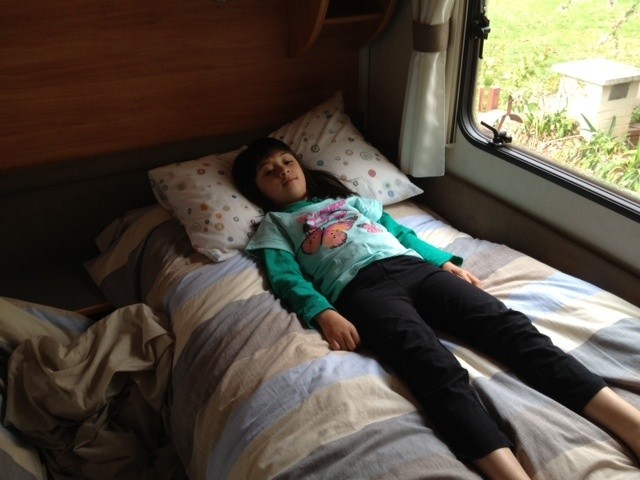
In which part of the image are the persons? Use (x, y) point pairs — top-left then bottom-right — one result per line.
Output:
(231, 136), (640, 480)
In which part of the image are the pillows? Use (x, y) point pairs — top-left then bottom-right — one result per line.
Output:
(145, 88), (426, 265)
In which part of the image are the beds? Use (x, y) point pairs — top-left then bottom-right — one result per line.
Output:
(1, 124), (640, 478)
